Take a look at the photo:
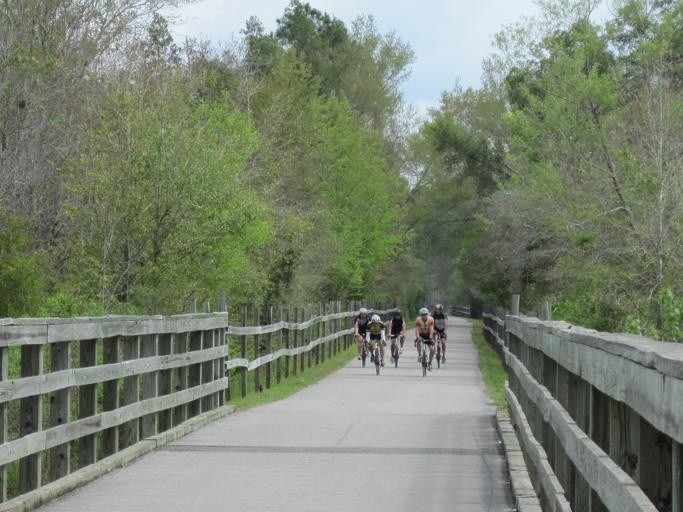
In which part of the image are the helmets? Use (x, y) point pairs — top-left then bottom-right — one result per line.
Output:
(359, 307), (367, 315)
(393, 309), (401, 317)
(418, 308), (429, 316)
(371, 314), (381, 323)
(435, 304), (443, 310)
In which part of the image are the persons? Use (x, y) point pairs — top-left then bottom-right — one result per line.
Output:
(353, 304), (448, 371)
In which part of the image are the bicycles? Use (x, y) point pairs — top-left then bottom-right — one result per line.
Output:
(389, 334), (404, 368)
(355, 334), (385, 375)
(416, 330), (444, 377)
